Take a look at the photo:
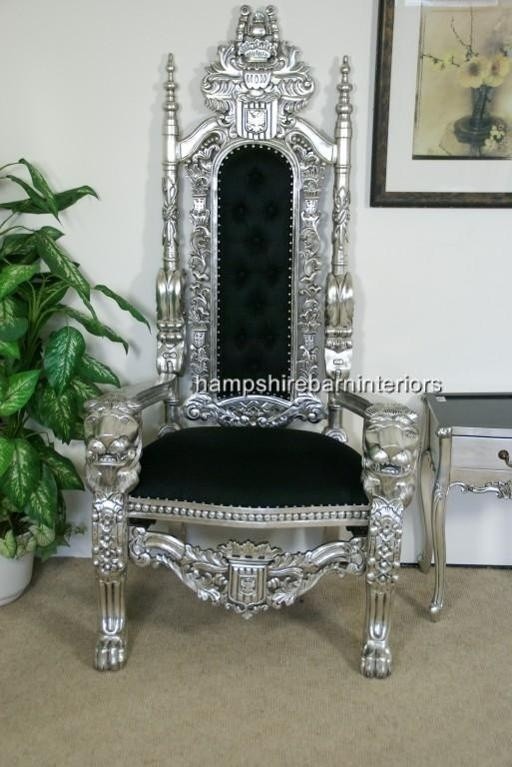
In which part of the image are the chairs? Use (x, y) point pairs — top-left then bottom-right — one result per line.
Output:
(83, 4), (423, 683)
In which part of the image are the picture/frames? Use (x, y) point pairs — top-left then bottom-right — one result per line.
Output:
(370, 0), (511, 208)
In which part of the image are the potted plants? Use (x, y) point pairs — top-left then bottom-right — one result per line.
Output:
(0, 157), (152, 612)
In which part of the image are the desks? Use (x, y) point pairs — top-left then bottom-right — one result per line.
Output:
(416, 390), (512, 617)
